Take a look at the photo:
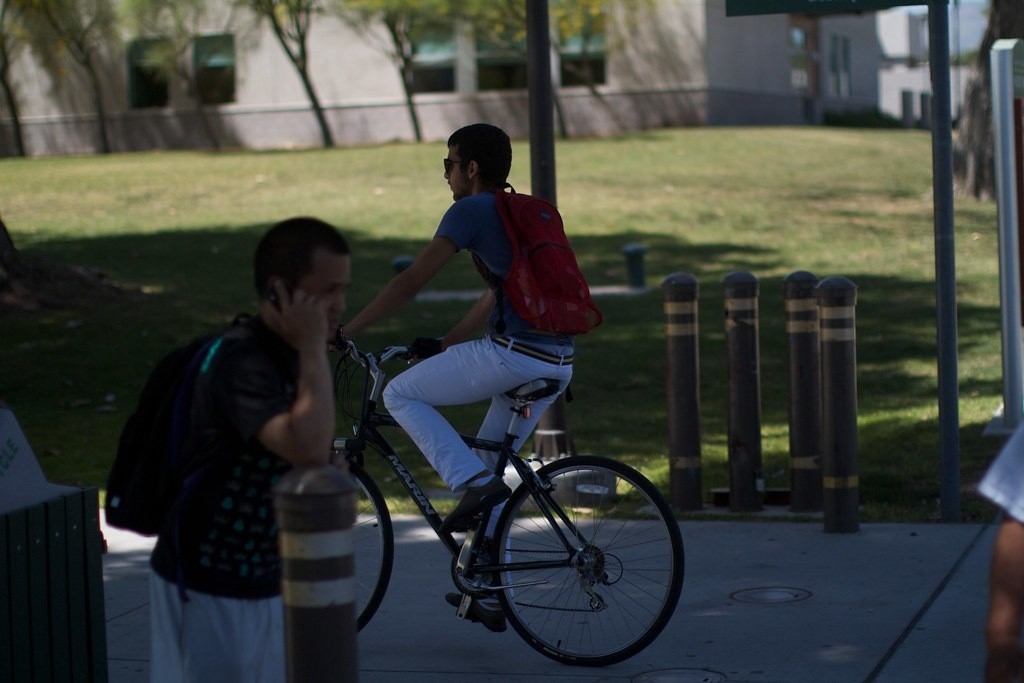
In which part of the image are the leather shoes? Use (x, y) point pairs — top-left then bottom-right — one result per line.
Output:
(439, 476), (512, 534)
(446, 593), (507, 632)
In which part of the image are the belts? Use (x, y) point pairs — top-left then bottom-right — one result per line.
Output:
(491, 335), (574, 365)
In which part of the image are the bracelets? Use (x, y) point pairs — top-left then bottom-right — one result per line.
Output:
(335, 323), (346, 349)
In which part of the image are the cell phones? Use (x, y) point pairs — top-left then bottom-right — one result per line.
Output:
(266, 284), (293, 313)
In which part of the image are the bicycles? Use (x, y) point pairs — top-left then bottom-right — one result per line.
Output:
(323, 325), (687, 671)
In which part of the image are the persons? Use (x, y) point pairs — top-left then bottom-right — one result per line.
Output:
(978, 414), (1024, 683)
(327, 124), (576, 634)
(149, 218), (353, 683)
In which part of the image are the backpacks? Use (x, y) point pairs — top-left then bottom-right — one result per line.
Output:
(104, 312), (253, 537)
(473, 184), (603, 336)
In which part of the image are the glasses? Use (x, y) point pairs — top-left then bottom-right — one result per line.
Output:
(444, 159), (465, 174)
